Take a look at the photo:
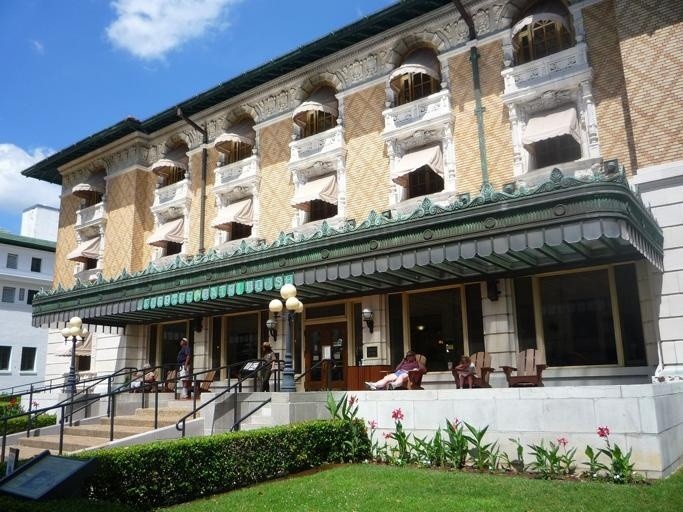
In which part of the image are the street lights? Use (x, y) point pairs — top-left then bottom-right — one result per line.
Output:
(270, 284), (306, 392)
(63, 314), (89, 392)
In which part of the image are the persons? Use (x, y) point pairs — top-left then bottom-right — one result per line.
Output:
(175, 337), (191, 399)
(134, 363), (155, 392)
(455, 356), (479, 389)
(365, 350), (420, 391)
(114, 367), (138, 392)
(260, 341), (276, 392)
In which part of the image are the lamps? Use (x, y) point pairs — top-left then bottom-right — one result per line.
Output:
(266, 319), (276, 339)
(361, 308), (374, 333)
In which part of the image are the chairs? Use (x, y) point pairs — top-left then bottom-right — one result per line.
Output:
(379, 354), (427, 390)
(150, 370), (176, 393)
(497, 348), (548, 387)
(450, 351), (494, 389)
(187, 370), (216, 400)
